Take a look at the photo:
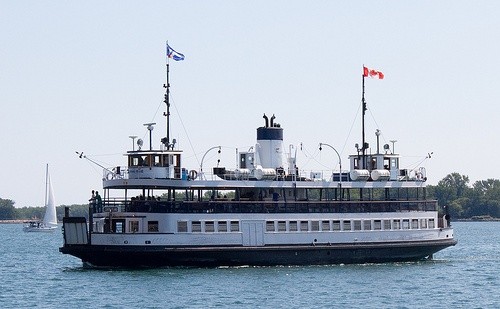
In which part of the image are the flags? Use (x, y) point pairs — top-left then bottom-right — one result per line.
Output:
(363, 66), (384, 80)
(167, 44), (185, 62)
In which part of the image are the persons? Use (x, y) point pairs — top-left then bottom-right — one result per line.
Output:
(270, 189), (281, 212)
(210, 194), (230, 212)
(130, 194), (162, 211)
(89, 189), (105, 215)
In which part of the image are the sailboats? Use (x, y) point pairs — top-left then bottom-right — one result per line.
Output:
(23, 162), (58, 231)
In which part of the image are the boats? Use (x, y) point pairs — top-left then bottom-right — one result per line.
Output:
(58, 40), (459, 266)
